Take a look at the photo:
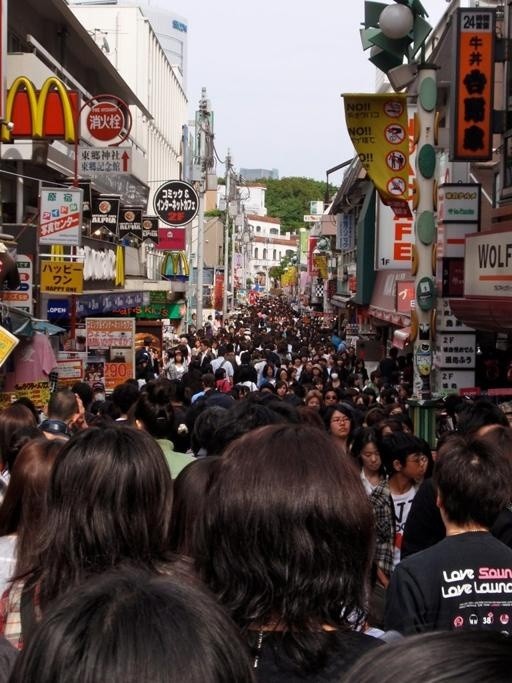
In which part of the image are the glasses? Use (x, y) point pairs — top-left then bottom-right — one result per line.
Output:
(324, 395), (339, 400)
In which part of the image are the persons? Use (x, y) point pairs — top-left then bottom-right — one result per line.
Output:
(2, 289), (512, 683)
